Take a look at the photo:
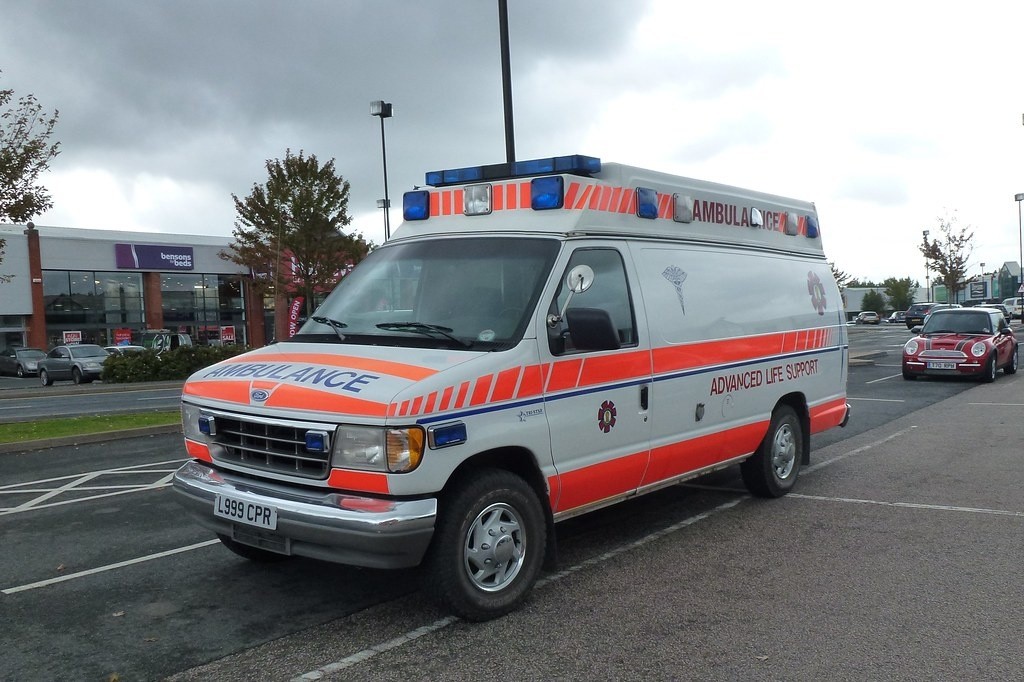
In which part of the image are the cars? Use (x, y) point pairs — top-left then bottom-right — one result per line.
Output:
(103, 345), (148, 370)
(921, 304), (963, 328)
(846, 318), (858, 325)
(970, 304), (1010, 327)
(855, 311), (880, 325)
(0, 347), (47, 378)
(878, 316), (889, 324)
(901, 307), (1019, 381)
(888, 310), (908, 323)
(39, 344), (114, 387)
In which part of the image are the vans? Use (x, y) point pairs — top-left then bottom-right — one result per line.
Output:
(960, 297), (1002, 307)
(1001, 297), (1024, 319)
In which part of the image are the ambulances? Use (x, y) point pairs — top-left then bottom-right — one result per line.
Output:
(174, 155), (853, 623)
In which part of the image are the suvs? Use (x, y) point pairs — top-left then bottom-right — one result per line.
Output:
(904, 302), (934, 331)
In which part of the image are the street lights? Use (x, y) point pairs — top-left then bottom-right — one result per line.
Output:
(1014, 193), (1024, 324)
(369, 99), (394, 245)
(980, 263), (986, 304)
(922, 230), (931, 303)
(377, 199), (392, 245)
